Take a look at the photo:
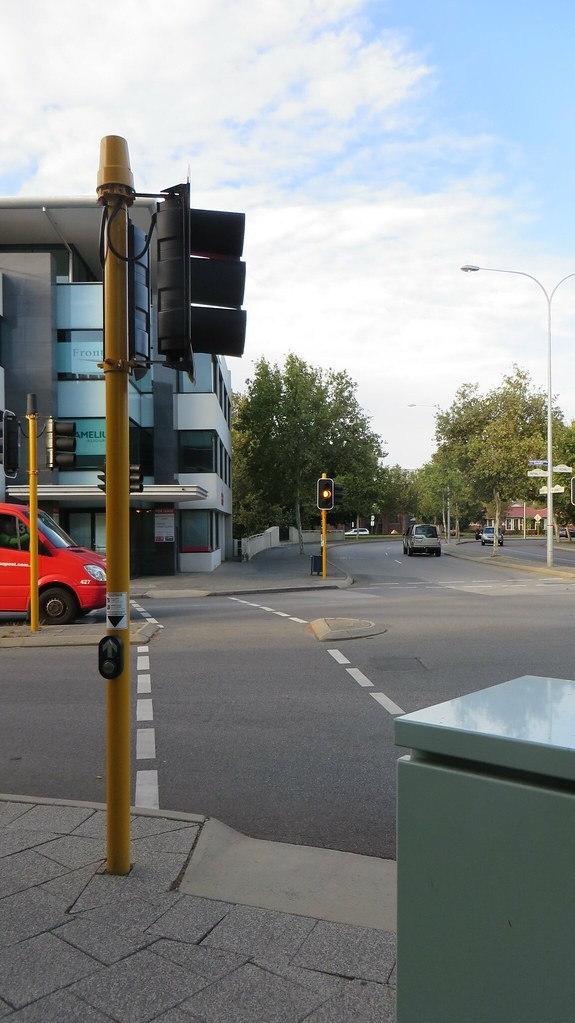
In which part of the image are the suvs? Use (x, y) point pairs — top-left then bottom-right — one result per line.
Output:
(403, 524), (441, 558)
(479, 527), (503, 547)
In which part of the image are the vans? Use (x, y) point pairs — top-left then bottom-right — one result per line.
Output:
(0, 502), (107, 625)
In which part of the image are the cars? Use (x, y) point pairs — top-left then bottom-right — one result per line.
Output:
(345, 528), (370, 534)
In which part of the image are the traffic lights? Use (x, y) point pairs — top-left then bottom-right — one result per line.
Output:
(318, 479), (333, 509)
(571, 476), (575, 507)
(150, 181), (246, 384)
(46, 414), (78, 472)
(130, 463), (143, 494)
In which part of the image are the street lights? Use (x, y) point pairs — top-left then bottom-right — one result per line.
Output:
(461, 261), (575, 570)
